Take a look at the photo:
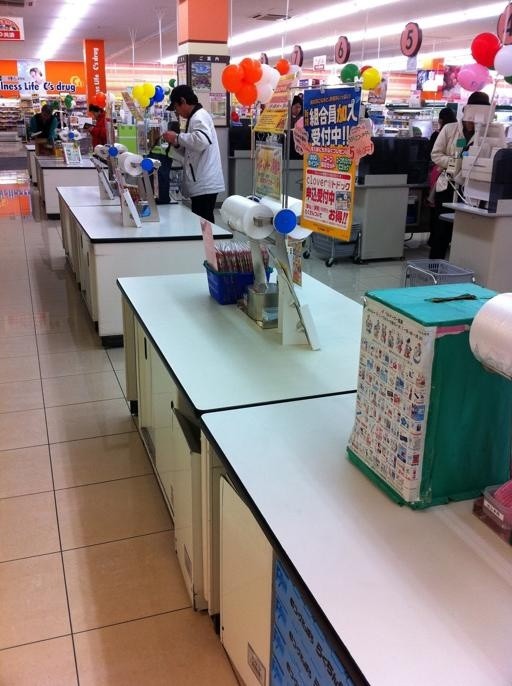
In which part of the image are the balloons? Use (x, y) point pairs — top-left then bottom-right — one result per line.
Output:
(51, 99), (59, 109)
(222, 54), (301, 109)
(493, 42), (511, 77)
(361, 67), (382, 90)
(42, 101), (47, 106)
(359, 64), (371, 75)
(454, 62), (488, 92)
(132, 82), (165, 109)
(341, 64), (360, 84)
(64, 95), (77, 109)
(470, 32), (501, 67)
(89, 92), (107, 107)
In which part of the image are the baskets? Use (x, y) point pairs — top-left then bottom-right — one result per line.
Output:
(402, 259), (474, 287)
(204, 260), (274, 305)
(312, 222), (360, 257)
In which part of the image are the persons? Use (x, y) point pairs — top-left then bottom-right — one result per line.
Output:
(31, 67), (44, 85)
(289, 96), (303, 129)
(427, 92), (491, 259)
(425, 109), (455, 247)
(163, 84), (226, 226)
(88, 104), (108, 150)
(31, 103), (58, 141)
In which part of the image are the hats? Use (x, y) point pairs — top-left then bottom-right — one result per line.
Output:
(462, 92), (491, 110)
(166, 85), (193, 111)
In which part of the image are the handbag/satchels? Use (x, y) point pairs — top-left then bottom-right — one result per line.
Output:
(436, 172), (462, 202)
(142, 152), (173, 203)
